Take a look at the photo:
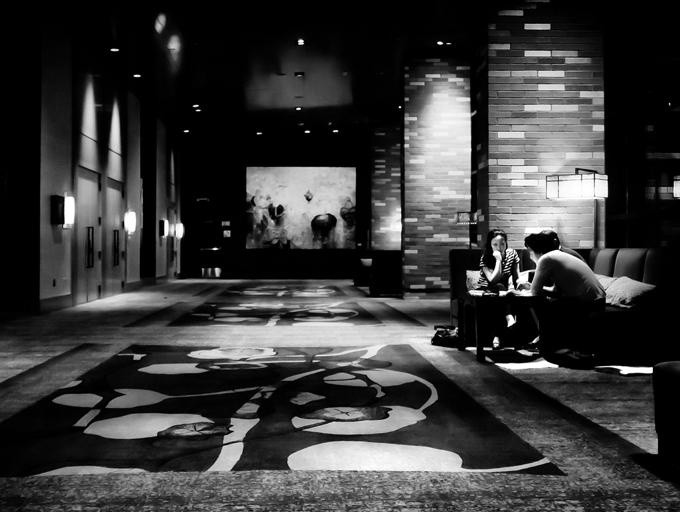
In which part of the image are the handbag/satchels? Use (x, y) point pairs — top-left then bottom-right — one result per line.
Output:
(431, 325), (459, 348)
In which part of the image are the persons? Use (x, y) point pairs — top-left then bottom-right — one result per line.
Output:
(524, 232), (606, 349)
(476, 229), (521, 349)
(541, 230), (587, 263)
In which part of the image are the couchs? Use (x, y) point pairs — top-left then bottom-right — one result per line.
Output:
(448, 246), (656, 360)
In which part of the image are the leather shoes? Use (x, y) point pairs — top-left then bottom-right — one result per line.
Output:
(493, 342), (500, 349)
(528, 338), (539, 349)
(508, 315), (516, 327)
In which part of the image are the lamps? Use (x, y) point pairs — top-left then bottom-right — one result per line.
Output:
(545, 167), (609, 249)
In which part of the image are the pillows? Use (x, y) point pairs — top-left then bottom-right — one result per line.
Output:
(516, 268), (556, 293)
(465, 269), (482, 291)
(602, 275), (657, 310)
(593, 273), (616, 290)
